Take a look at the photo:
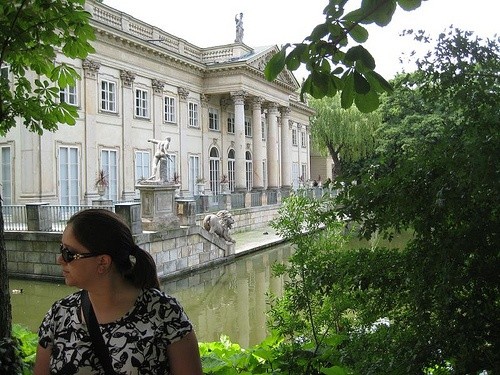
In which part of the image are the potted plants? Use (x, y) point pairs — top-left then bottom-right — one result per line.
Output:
(317, 175), (323, 187)
(297, 176), (304, 189)
(171, 173), (182, 197)
(195, 175), (207, 193)
(220, 175), (229, 192)
(93, 169), (110, 196)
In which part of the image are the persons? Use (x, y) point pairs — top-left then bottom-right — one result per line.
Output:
(33, 209), (202, 375)
(234, 12), (245, 43)
(312, 180), (319, 187)
(147, 137), (173, 180)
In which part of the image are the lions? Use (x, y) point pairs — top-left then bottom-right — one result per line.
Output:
(204, 210), (236, 244)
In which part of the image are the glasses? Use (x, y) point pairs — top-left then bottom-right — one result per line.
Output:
(58, 243), (97, 264)
